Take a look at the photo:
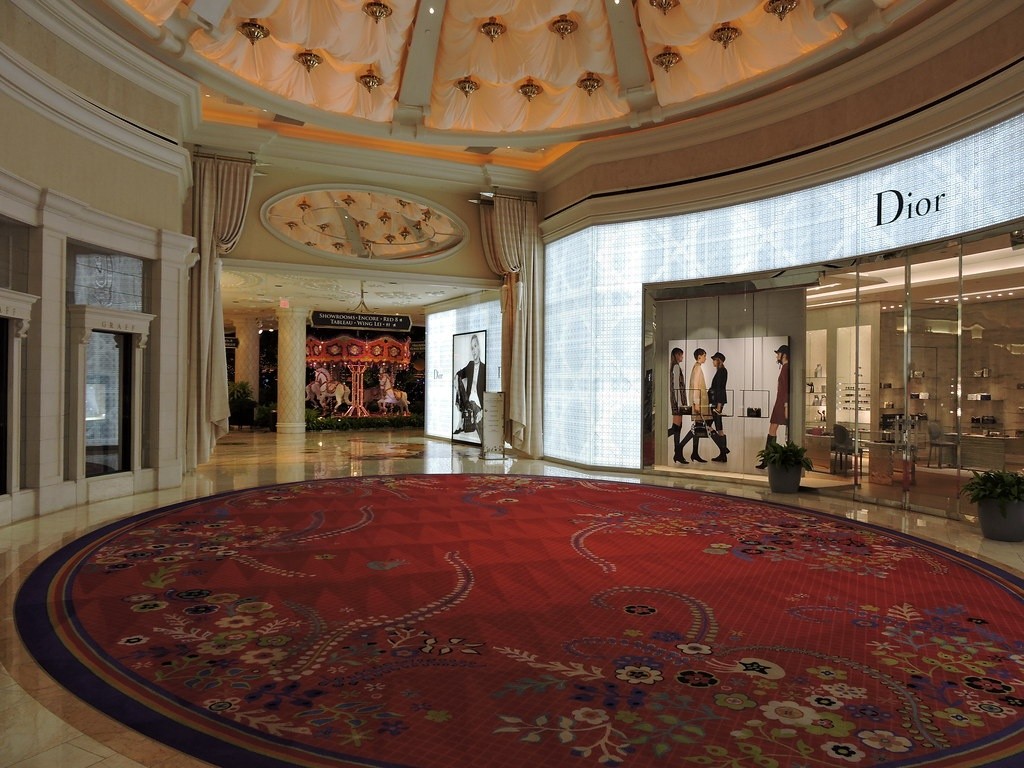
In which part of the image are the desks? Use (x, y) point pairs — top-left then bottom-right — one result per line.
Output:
(944, 432), (1024, 472)
(864, 440), (917, 486)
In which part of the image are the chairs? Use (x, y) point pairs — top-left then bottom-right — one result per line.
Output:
(926, 420), (955, 468)
(833, 424), (863, 478)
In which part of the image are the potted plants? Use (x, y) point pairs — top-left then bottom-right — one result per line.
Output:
(959, 469), (1024, 542)
(755, 441), (815, 494)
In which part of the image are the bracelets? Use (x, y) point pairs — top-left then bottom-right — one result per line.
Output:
(784, 404), (788, 407)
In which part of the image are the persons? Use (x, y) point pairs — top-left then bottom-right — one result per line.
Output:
(755, 345), (789, 469)
(453, 335), (486, 435)
(668, 347), (730, 464)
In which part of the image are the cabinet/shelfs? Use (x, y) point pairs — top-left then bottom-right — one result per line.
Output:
(805, 377), (826, 426)
(838, 382), (871, 411)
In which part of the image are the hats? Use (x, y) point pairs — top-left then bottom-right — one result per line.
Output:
(711, 352), (725, 362)
(775, 345), (791, 356)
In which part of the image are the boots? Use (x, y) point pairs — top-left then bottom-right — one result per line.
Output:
(672, 423), (689, 464)
(680, 430), (694, 447)
(691, 435), (708, 463)
(667, 423), (683, 437)
(708, 430), (730, 454)
(755, 434), (777, 469)
(711, 435), (727, 462)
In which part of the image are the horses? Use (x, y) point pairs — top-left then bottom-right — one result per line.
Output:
(305, 365), (353, 417)
(363, 371), (412, 416)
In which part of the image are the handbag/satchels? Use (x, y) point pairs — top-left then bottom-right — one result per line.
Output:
(691, 413), (711, 438)
(747, 408), (761, 418)
